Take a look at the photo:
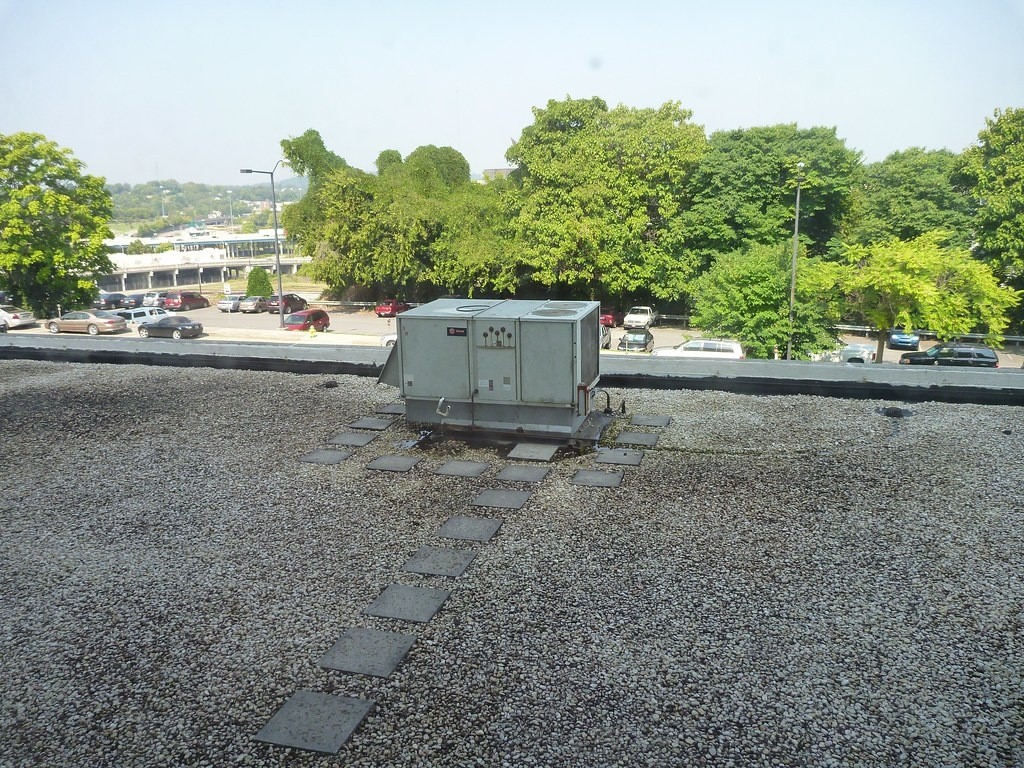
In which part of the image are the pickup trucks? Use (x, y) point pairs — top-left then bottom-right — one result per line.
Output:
(623, 304), (660, 329)
(375, 298), (411, 317)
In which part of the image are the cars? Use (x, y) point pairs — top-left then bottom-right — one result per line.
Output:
(889, 326), (920, 350)
(165, 291), (210, 311)
(92, 292), (128, 308)
(137, 315), (205, 340)
(142, 291), (167, 308)
(217, 295), (248, 312)
(808, 344), (879, 366)
(0, 304), (38, 331)
(239, 295), (270, 313)
(651, 337), (745, 361)
(616, 328), (655, 353)
(599, 323), (612, 349)
(899, 342), (1000, 370)
(119, 293), (145, 309)
(44, 309), (128, 335)
(267, 293), (308, 314)
(600, 305), (624, 328)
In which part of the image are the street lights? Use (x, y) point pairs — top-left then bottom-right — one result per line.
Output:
(239, 160), (286, 328)
(786, 160), (804, 364)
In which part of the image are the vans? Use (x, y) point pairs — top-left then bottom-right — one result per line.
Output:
(118, 307), (169, 327)
(279, 309), (331, 333)
